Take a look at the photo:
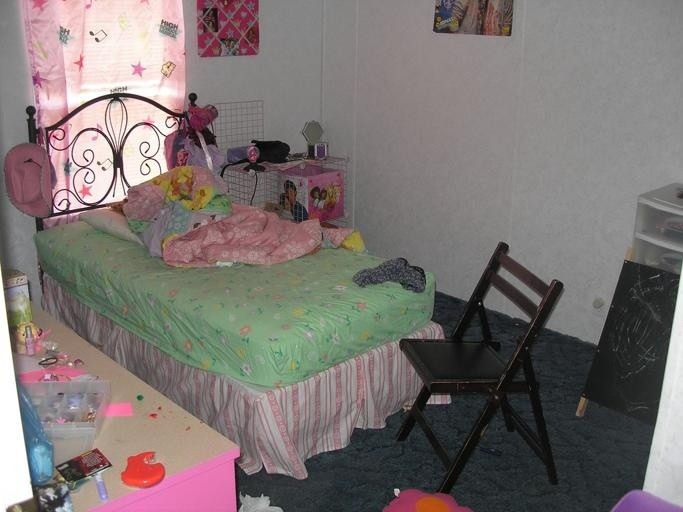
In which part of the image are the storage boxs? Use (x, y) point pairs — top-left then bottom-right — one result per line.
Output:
(629, 181), (682, 277)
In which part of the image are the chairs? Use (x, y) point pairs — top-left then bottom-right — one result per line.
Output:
(398, 240), (565, 494)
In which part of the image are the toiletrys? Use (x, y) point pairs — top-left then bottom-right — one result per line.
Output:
(25, 323), (37, 359)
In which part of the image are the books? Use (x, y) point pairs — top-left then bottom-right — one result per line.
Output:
(55, 448), (111, 483)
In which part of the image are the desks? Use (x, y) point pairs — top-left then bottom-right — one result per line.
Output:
(9, 289), (241, 512)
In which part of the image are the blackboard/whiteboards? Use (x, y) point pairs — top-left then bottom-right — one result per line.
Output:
(581, 260), (680, 425)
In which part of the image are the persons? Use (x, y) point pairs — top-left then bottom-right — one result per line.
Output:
(277, 179), (307, 223)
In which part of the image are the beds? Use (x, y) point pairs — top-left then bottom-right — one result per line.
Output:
(23, 92), (445, 476)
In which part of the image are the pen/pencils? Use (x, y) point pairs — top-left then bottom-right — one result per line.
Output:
(457, 440), (502, 456)
(95, 474), (108, 500)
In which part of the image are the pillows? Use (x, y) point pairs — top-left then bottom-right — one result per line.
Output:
(74, 205), (148, 246)
